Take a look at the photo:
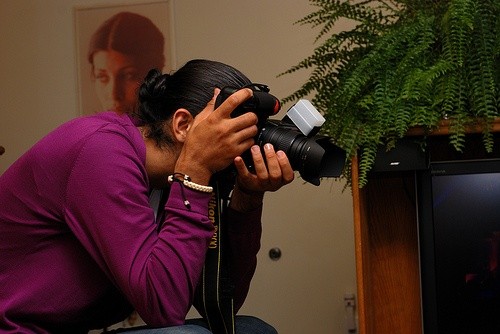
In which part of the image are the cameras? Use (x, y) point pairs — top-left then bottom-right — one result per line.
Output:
(217, 85), (347, 186)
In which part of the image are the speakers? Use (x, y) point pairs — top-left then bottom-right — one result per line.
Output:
(358, 134), (426, 174)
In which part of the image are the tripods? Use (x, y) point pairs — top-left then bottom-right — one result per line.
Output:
(415, 155), (500, 334)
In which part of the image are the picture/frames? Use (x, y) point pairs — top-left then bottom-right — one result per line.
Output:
(72, 0), (175, 116)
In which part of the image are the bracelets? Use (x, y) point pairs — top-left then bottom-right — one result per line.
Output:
(235, 177), (261, 196)
(168, 173), (213, 208)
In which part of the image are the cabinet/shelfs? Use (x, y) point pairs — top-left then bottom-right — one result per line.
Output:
(351, 116), (500, 334)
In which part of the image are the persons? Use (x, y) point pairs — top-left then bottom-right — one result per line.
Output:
(0, 59), (295, 334)
